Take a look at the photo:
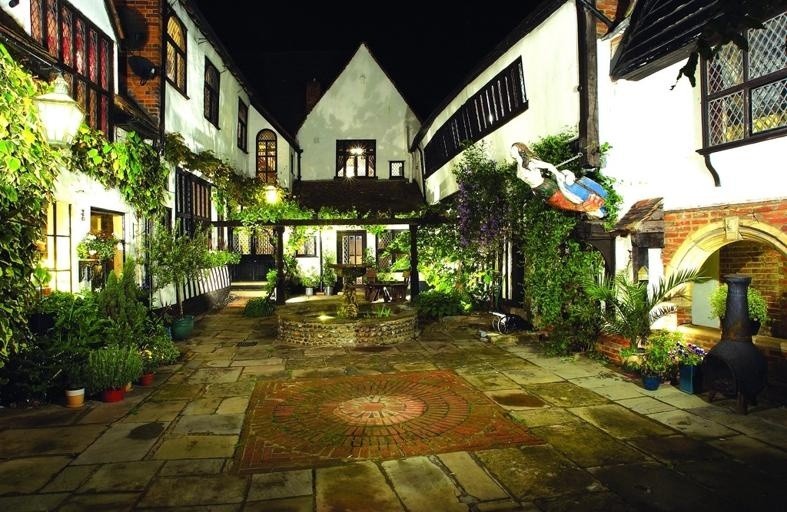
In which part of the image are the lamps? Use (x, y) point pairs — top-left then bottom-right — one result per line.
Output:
(34, 65), (87, 150)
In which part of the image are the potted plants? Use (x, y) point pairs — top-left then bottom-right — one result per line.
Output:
(266, 256), (338, 301)
(61, 357), (88, 409)
(582, 264), (715, 396)
(138, 348), (158, 388)
(129, 216), (243, 342)
(87, 342), (143, 403)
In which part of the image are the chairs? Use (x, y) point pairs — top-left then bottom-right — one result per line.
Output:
(362, 268), (411, 302)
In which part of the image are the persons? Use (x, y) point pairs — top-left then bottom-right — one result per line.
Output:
(510, 142), (606, 219)
